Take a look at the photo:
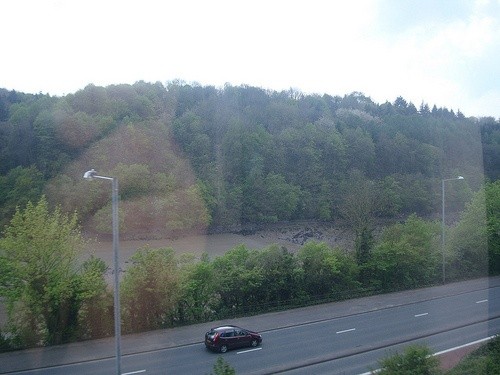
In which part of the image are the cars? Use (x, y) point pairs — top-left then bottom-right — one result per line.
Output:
(204, 325), (262, 353)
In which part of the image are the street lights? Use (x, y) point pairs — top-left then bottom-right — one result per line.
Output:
(83, 168), (124, 374)
(440, 176), (465, 283)
